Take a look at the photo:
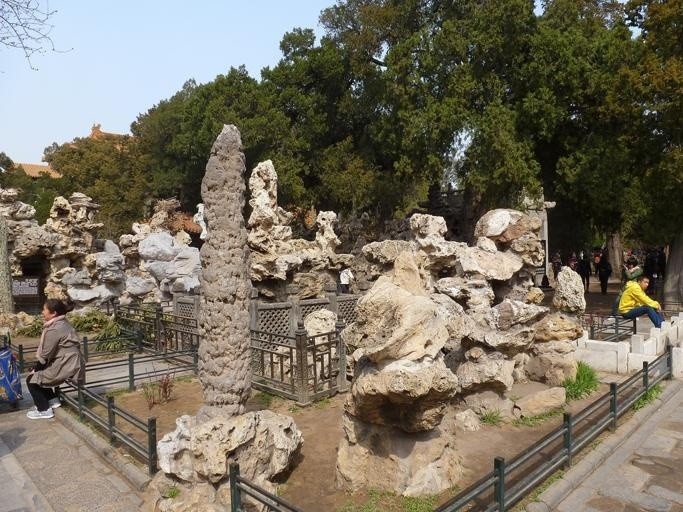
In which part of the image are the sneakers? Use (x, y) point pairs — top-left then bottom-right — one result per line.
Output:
(26, 397), (61, 419)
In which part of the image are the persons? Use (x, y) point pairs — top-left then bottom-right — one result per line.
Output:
(656, 246), (666, 280)
(25, 298), (86, 420)
(595, 255), (612, 295)
(618, 274), (676, 329)
(647, 248), (656, 278)
(608, 257), (644, 316)
(578, 254), (592, 294)
